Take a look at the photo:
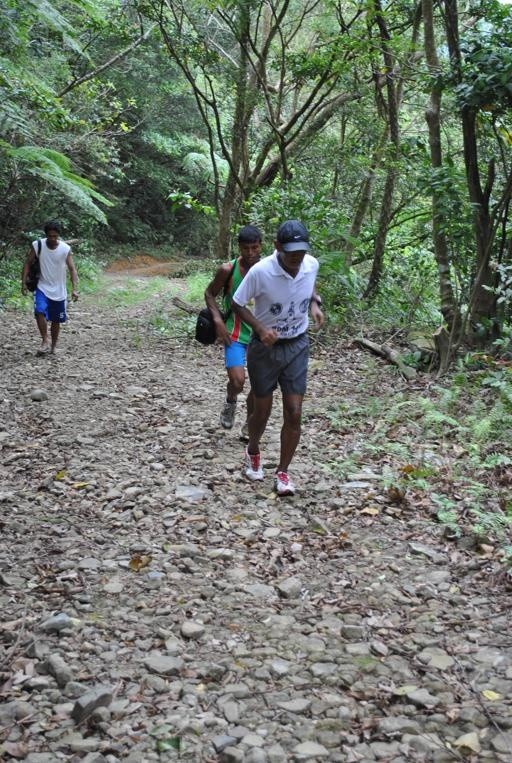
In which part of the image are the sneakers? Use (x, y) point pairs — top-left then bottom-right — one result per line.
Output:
(239, 420), (250, 442)
(39, 341), (56, 357)
(244, 444), (264, 482)
(220, 395), (237, 429)
(274, 470), (295, 496)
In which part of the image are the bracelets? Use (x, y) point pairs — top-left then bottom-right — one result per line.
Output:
(311, 294), (322, 306)
(23, 280), (27, 285)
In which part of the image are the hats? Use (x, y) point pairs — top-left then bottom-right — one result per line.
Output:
(277, 220), (310, 253)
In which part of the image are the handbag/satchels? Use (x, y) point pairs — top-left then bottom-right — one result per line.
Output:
(25, 258), (41, 292)
(194, 306), (225, 346)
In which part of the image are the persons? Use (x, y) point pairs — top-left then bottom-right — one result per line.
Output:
(230, 219), (325, 496)
(204, 223), (263, 442)
(22, 220), (80, 354)
